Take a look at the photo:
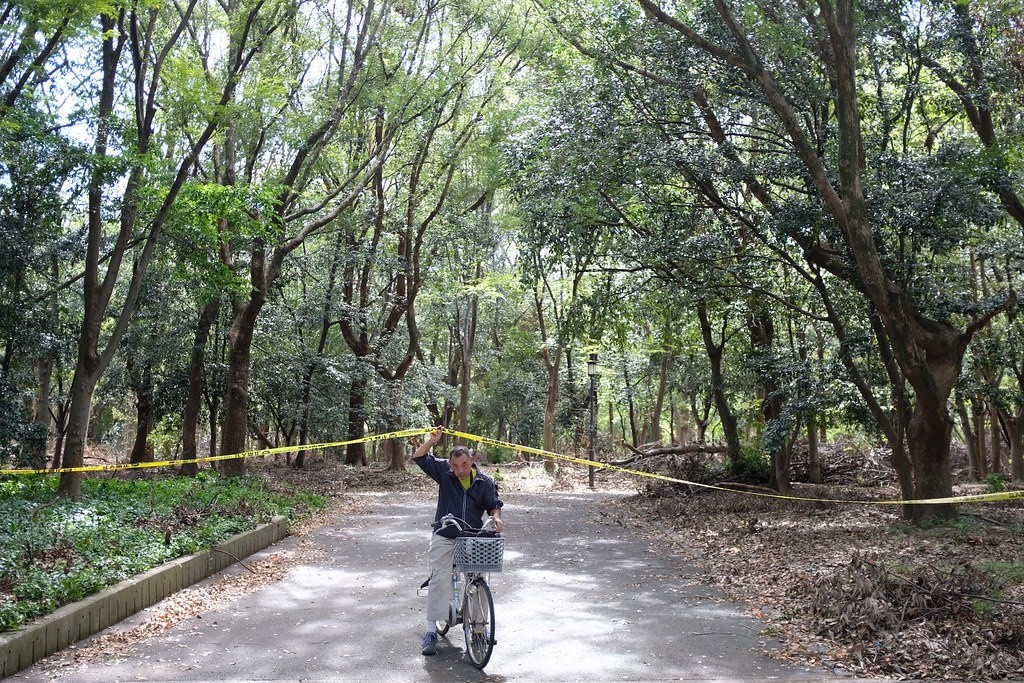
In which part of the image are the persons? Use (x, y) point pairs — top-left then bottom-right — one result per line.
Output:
(412, 425), (505, 655)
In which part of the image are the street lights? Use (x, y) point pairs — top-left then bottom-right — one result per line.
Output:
(586, 355), (597, 489)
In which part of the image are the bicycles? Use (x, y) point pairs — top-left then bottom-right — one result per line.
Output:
(416, 513), (506, 670)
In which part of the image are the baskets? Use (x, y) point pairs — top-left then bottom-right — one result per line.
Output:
(454, 537), (505, 573)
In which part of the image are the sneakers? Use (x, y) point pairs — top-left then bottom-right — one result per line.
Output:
(472, 633), (497, 645)
(421, 631), (438, 654)
